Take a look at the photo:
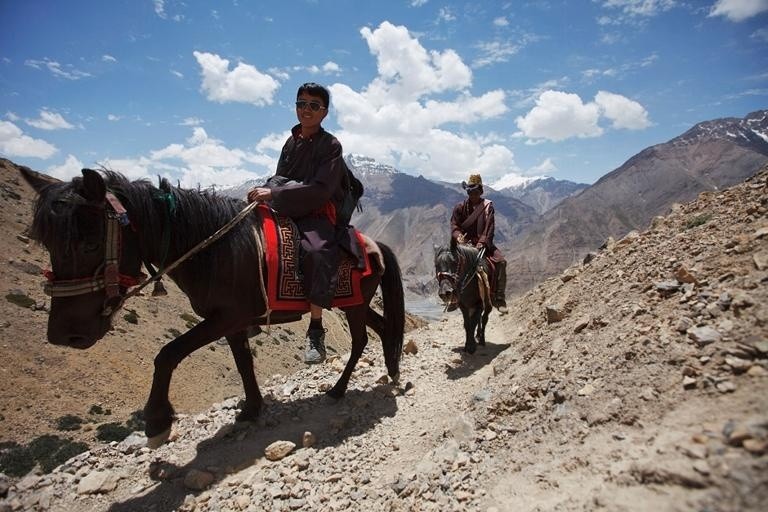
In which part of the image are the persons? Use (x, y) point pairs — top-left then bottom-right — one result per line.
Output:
(447, 188), (509, 313)
(216, 83), (350, 364)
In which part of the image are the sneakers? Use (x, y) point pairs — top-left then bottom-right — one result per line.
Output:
(447, 303), (459, 312)
(492, 296), (508, 313)
(303, 328), (329, 364)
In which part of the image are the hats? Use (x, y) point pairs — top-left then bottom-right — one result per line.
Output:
(462, 174), (483, 195)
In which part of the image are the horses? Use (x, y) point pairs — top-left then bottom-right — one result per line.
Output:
(433, 234), (496, 354)
(18, 166), (405, 450)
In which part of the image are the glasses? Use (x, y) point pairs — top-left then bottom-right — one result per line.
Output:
(294, 98), (325, 112)
